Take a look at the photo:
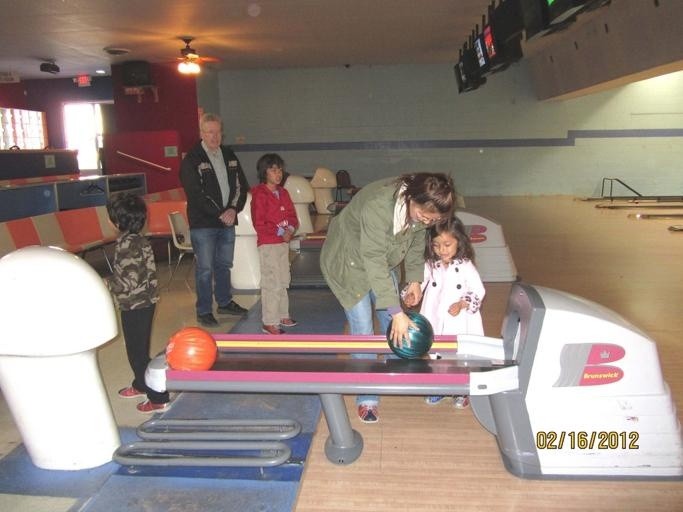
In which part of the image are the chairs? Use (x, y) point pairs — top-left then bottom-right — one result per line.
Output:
(0, 188), (184, 262)
(146, 201), (187, 264)
(335, 170), (354, 202)
(166, 211), (194, 298)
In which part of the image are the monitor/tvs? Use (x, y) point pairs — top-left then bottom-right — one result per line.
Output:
(457, 58), (473, 89)
(472, 33), (495, 70)
(481, 22), (510, 67)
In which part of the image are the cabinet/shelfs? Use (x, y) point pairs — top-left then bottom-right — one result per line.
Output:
(0, 172), (147, 224)
(0, 149), (80, 181)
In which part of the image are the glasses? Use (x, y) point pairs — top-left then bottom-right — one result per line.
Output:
(418, 211), (442, 222)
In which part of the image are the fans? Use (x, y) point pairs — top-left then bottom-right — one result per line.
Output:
(153, 49), (225, 76)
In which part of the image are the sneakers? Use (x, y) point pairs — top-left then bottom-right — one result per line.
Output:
(358, 402), (379, 424)
(217, 299), (248, 314)
(262, 324), (287, 335)
(136, 399), (170, 413)
(279, 318), (297, 326)
(423, 395), (445, 404)
(118, 385), (148, 398)
(197, 311), (218, 327)
(452, 394), (470, 409)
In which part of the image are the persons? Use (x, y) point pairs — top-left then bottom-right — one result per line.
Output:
(399, 216), (486, 409)
(250, 153), (301, 336)
(319, 171), (457, 424)
(101, 192), (172, 415)
(178, 113), (249, 327)
